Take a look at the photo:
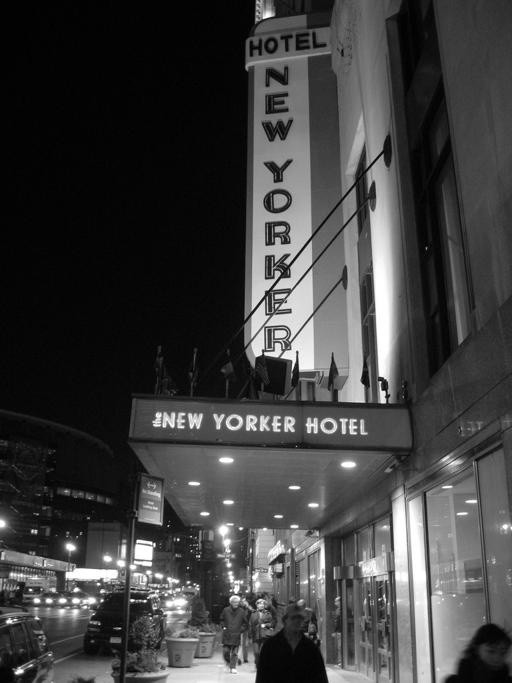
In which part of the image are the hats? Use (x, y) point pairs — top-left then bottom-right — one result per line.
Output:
(282, 603), (306, 620)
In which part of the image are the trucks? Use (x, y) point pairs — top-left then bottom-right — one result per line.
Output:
(22, 577), (50, 606)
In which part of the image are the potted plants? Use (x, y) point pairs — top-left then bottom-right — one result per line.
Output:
(184, 600), (216, 658)
(110, 617), (169, 683)
(164, 629), (199, 667)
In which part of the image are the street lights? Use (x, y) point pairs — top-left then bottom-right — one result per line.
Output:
(66, 543), (76, 592)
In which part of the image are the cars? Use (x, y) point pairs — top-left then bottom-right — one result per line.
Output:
(33, 592), (107, 609)
(158, 591), (197, 610)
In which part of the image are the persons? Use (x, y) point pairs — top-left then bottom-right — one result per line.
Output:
(444, 622), (512, 683)
(220, 595), (328, 683)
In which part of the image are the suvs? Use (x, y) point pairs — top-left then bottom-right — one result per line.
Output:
(0, 603), (55, 682)
(83, 588), (167, 657)
